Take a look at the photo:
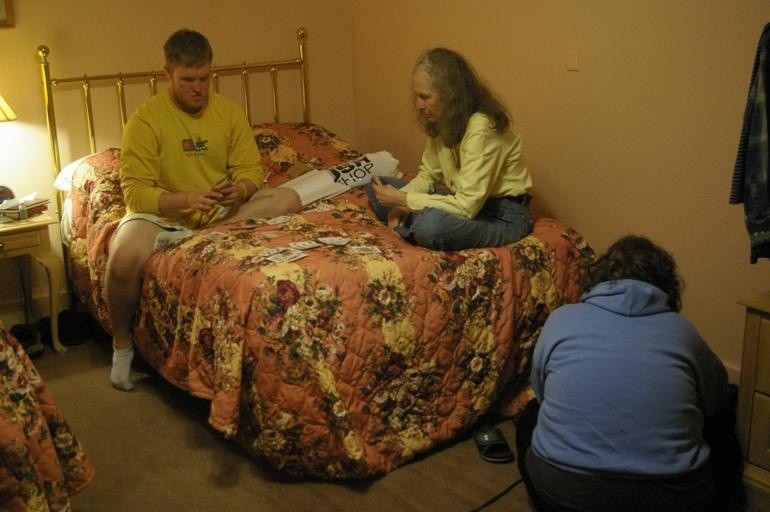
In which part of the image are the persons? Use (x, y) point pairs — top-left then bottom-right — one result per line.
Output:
(517, 235), (747, 512)
(367, 48), (534, 252)
(105, 27), (302, 390)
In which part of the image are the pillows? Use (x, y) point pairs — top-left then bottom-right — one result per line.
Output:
(52, 152), (105, 193)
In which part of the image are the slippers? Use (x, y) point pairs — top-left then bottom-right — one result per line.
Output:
(474, 424), (515, 463)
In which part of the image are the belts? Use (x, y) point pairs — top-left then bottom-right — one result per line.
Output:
(505, 193), (532, 207)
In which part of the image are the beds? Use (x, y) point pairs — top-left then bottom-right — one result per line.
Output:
(35, 27), (580, 460)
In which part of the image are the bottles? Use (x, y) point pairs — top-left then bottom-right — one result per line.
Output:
(19, 202), (27, 219)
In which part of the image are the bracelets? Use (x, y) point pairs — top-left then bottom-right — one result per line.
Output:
(239, 182), (248, 200)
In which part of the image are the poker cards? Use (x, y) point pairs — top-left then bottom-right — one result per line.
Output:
(266, 215), (290, 225)
(371, 172), (384, 186)
(210, 175), (233, 204)
(258, 235), (382, 266)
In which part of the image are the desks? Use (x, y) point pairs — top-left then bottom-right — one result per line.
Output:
(0, 211), (71, 357)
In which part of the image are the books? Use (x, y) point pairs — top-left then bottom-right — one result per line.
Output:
(0, 197), (51, 219)
(279, 169), (352, 212)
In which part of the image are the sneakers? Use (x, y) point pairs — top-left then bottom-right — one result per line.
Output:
(39, 309), (86, 345)
(10, 323), (46, 360)
